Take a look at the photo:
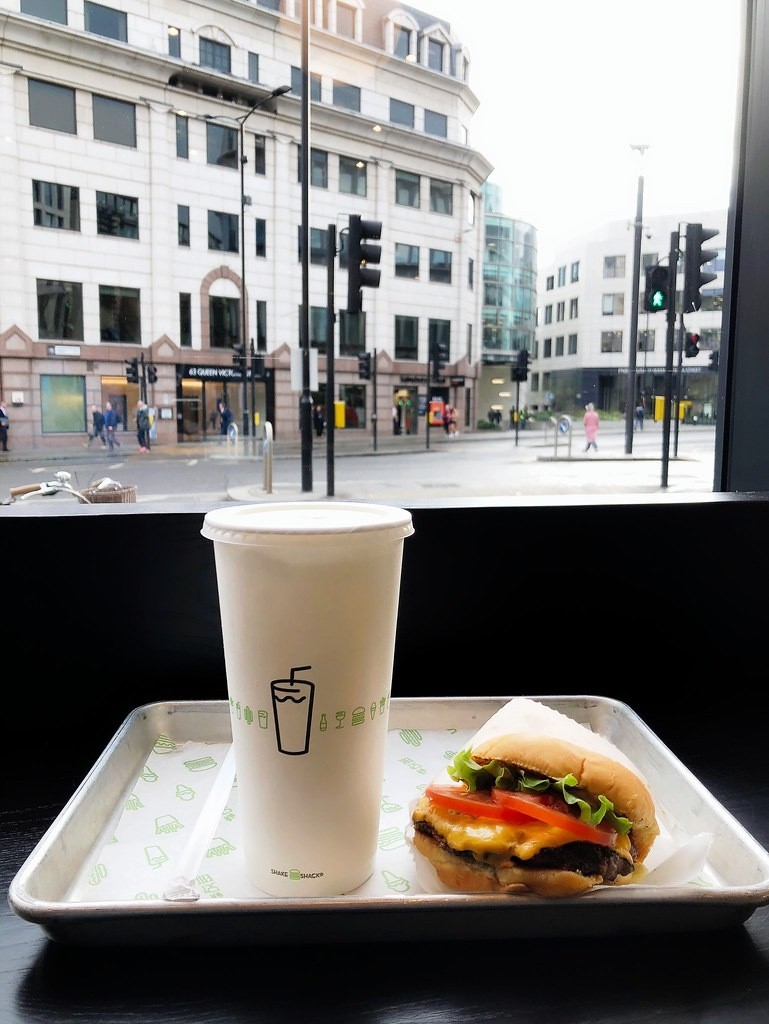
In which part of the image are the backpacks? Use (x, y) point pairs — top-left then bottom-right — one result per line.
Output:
(636, 410), (644, 419)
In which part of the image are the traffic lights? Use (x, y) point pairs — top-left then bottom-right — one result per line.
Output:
(645, 266), (671, 312)
(124, 357), (138, 384)
(148, 365), (157, 384)
(333, 400), (345, 428)
(684, 332), (701, 358)
(359, 352), (370, 381)
(433, 343), (447, 376)
(652, 396), (665, 423)
(233, 344), (244, 368)
(520, 350), (532, 382)
(347, 214), (382, 314)
(709, 351), (718, 372)
(685, 224), (719, 314)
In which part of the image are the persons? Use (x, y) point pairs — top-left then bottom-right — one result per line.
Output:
(510, 405), (529, 430)
(0, 401), (10, 451)
(634, 401), (644, 431)
(443, 404), (459, 438)
(583, 403), (600, 452)
(82, 405), (109, 449)
(315, 404), (327, 436)
(488, 406), (502, 425)
(392, 400), (417, 435)
(345, 400), (358, 428)
(134, 400), (154, 454)
(218, 402), (233, 445)
(103, 401), (123, 452)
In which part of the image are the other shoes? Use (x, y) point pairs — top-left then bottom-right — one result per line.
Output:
(140, 446), (150, 453)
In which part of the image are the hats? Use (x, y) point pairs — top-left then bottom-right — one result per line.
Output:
(585, 402), (594, 411)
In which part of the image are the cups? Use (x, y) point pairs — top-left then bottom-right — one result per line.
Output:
(201, 502), (415, 898)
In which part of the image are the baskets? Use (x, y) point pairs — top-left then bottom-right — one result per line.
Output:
(78, 484), (137, 504)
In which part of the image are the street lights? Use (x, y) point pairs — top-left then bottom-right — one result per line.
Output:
(195, 84), (293, 453)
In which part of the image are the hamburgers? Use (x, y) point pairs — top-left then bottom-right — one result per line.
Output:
(410, 734), (660, 898)
